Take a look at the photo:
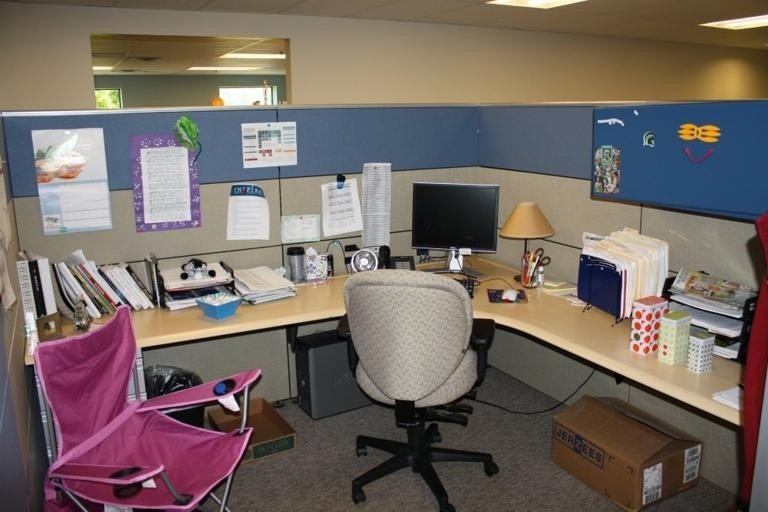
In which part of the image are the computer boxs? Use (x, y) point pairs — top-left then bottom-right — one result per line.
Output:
(294, 330), (373, 420)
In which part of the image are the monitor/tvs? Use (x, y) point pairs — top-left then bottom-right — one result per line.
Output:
(410, 181), (501, 280)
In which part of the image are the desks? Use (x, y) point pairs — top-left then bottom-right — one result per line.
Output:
(21, 248), (746, 512)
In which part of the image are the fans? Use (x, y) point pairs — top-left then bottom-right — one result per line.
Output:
(350, 250), (378, 274)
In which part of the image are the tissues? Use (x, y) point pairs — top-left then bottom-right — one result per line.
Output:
(302, 247), (328, 284)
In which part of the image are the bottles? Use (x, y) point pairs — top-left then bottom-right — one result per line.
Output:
(285, 245), (305, 285)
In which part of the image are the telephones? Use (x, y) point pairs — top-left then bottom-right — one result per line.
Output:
(378, 246), (415, 270)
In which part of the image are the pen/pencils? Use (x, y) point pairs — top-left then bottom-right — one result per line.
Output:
(522, 250), (540, 288)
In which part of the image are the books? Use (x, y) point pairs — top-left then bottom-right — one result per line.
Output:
(15, 247), (156, 336)
(713, 384), (743, 410)
(577, 227), (756, 359)
(232, 264), (298, 306)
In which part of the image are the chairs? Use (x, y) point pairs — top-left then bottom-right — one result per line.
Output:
(36, 302), (263, 512)
(335, 266), (500, 511)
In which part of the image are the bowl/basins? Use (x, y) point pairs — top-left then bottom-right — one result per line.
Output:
(195, 292), (242, 320)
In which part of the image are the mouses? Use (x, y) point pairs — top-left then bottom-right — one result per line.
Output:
(501, 288), (521, 302)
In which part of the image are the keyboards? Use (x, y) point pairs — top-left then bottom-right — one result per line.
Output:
(452, 278), (475, 299)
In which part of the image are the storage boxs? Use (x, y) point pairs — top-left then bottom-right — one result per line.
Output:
(207, 395), (298, 462)
(550, 393), (706, 512)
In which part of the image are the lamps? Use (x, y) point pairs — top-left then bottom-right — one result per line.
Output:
(501, 200), (555, 281)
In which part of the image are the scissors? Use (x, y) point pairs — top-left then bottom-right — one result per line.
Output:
(536, 248), (550, 266)
